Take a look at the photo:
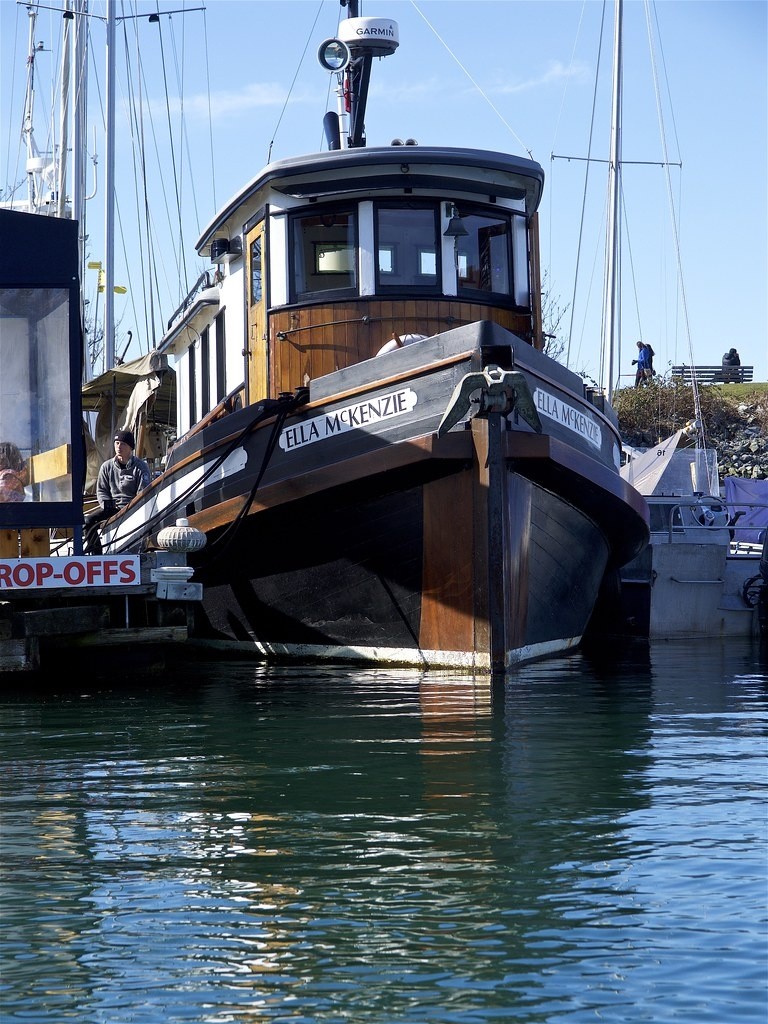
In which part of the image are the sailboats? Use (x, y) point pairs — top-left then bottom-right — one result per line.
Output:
(0, 0), (209, 675)
(547, 0), (768, 640)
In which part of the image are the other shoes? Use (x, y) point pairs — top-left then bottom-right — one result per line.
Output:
(82, 542), (102, 555)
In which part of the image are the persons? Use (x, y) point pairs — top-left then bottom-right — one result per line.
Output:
(82, 431), (151, 555)
(0, 442), (28, 502)
(632, 341), (651, 387)
(722, 348), (740, 384)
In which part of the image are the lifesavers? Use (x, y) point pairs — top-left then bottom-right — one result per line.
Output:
(377, 334), (431, 357)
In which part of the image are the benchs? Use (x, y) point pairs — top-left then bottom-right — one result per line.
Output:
(671, 366), (753, 385)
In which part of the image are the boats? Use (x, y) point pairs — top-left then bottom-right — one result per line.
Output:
(48, 0), (652, 674)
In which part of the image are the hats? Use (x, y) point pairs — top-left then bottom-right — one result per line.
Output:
(113, 432), (135, 450)
